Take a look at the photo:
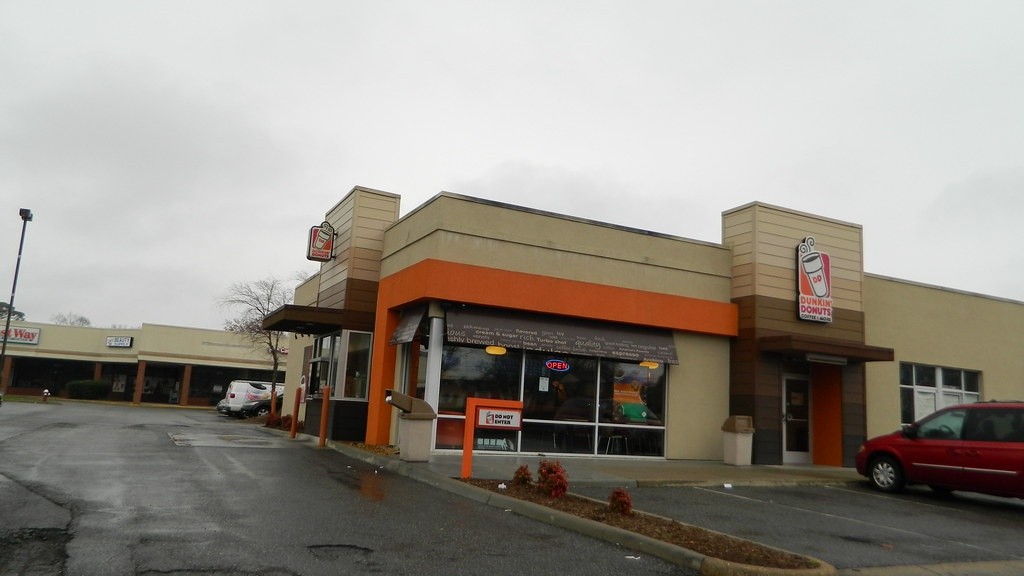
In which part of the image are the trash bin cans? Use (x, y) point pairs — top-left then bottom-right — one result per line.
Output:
(398, 397), (437, 462)
(720, 415), (755, 466)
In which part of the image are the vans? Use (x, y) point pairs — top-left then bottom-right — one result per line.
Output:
(224, 379), (284, 418)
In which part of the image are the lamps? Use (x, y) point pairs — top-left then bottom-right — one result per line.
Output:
(485, 346), (508, 355)
(638, 361), (660, 371)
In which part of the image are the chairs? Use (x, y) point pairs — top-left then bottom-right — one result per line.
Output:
(1003, 422), (1022, 441)
(981, 419), (998, 442)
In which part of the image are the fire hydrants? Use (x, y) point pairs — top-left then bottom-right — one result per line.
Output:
(42, 389), (50, 401)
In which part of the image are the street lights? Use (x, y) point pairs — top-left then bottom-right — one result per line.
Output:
(0, 208), (33, 375)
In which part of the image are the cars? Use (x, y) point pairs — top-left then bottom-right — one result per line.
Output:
(613, 402), (662, 429)
(242, 394), (283, 417)
(855, 401), (1024, 501)
(217, 399), (229, 415)
